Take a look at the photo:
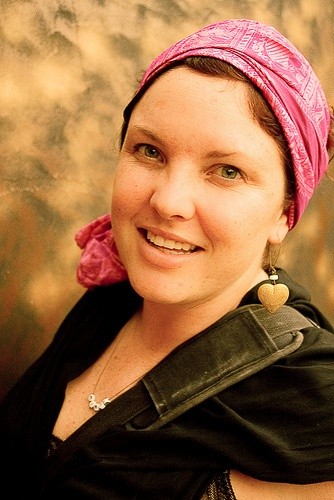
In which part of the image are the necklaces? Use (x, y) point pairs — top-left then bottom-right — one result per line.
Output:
(88, 321), (189, 411)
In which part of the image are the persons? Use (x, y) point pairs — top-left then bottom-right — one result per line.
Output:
(0, 20), (334, 500)
